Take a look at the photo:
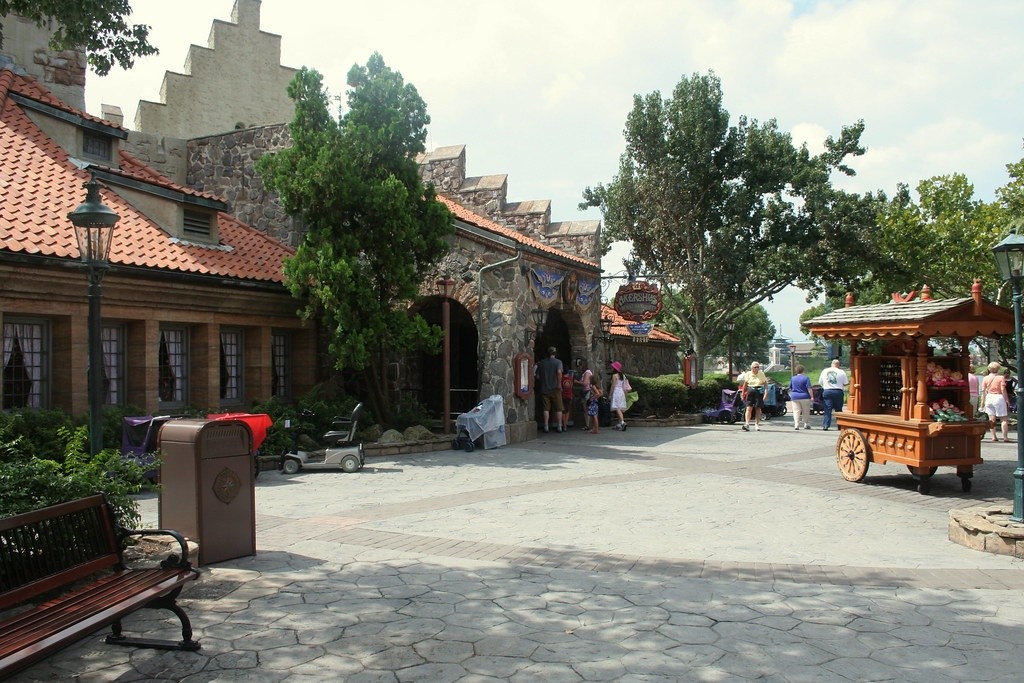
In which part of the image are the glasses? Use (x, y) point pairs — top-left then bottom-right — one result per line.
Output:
(752, 367), (760, 368)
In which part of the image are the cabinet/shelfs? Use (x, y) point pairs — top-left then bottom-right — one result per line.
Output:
(854, 355), (966, 420)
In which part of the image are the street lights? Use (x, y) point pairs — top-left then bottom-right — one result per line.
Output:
(725, 318), (735, 381)
(66, 171), (121, 460)
(991, 224), (1024, 524)
(986, 340), (991, 375)
(789, 344), (796, 376)
(436, 275), (458, 434)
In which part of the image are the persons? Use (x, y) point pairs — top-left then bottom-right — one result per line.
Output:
(561, 364), (572, 431)
(819, 359), (849, 430)
(741, 361), (769, 431)
(574, 360), (593, 430)
(607, 361), (627, 431)
(1003, 369), (1018, 412)
(980, 371), (988, 377)
(536, 346), (563, 433)
(981, 361), (1010, 442)
(588, 374), (603, 434)
(789, 366), (813, 430)
(968, 364), (979, 418)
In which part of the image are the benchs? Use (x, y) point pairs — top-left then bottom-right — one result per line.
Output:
(0, 494), (201, 683)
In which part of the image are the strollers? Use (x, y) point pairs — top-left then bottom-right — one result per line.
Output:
(106, 415), (191, 494)
(702, 388), (742, 425)
(810, 385), (826, 414)
(450, 395), (505, 452)
(737, 383), (790, 421)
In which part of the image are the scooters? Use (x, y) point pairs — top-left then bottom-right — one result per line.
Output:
(278, 402), (365, 474)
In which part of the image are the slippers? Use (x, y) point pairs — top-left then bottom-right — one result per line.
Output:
(581, 426), (590, 431)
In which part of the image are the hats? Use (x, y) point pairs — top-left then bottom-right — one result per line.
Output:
(610, 361), (623, 372)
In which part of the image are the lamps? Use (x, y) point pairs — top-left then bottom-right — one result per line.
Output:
(592, 315), (613, 349)
(522, 303), (549, 347)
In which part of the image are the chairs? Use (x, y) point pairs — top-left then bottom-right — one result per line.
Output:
(322, 401), (364, 449)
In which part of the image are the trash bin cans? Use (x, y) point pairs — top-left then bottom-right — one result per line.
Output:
(157, 418), (257, 567)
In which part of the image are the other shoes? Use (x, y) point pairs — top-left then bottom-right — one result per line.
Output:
(558, 427), (562, 431)
(794, 426), (799, 430)
(590, 430), (599, 434)
(621, 422), (627, 430)
(742, 424), (750, 431)
(611, 424), (620, 429)
(543, 426), (549, 432)
(755, 425), (761, 431)
(804, 425), (811, 429)
(823, 427), (828, 431)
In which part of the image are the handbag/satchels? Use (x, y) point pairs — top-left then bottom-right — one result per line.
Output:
(622, 379), (632, 393)
(572, 385), (585, 398)
(585, 390), (591, 403)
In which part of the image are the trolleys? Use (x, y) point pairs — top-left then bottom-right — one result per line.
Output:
(207, 412), (273, 478)
